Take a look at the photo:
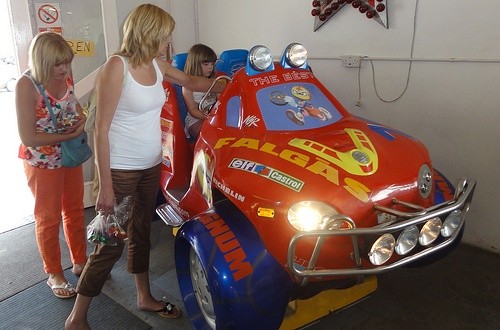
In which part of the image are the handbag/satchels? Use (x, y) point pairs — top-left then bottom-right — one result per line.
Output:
(60, 132), (92, 168)
(87, 196), (134, 247)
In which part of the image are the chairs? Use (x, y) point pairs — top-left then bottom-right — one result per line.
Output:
(170, 52), (197, 143)
(215, 49), (249, 77)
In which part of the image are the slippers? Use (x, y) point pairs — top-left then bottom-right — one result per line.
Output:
(154, 300), (181, 319)
(47, 281), (77, 298)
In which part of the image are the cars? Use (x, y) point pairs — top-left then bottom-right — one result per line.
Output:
(0, 56), (19, 93)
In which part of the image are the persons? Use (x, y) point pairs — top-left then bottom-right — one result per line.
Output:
(182, 43), (217, 140)
(14, 31), (90, 298)
(64, 4), (228, 330)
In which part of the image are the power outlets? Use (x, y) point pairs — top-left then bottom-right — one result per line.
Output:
(340, 55), (361, 68)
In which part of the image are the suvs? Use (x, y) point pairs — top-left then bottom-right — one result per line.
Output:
(155, 43), (478, 330)
